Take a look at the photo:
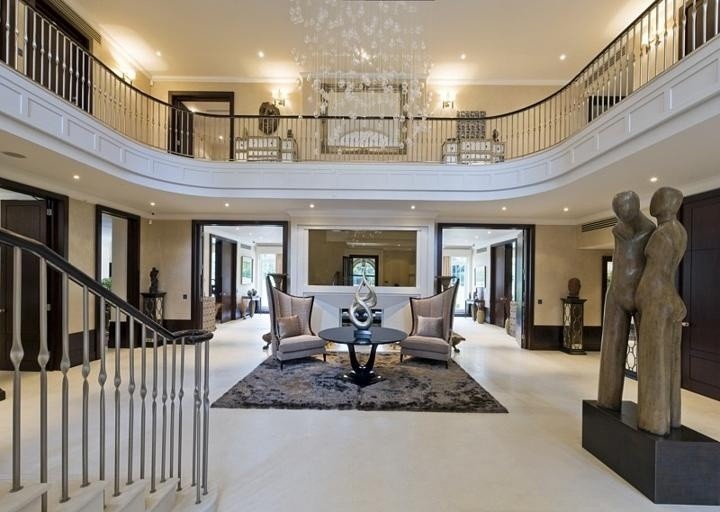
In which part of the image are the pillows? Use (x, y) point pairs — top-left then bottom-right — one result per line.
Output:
(276, 315), (302, 337)
(414, 314), (444, 337)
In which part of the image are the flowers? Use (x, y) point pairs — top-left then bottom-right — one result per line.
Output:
(248, 289), (257, 300)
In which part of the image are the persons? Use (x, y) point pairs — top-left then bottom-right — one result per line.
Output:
(149, 267), (159, 293)
(598, 190), (650, 412)
(632, 186), (689, 437)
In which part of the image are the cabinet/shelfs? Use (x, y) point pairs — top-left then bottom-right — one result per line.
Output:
(140, 291), (167, 345)
(560, 297), (587, 355)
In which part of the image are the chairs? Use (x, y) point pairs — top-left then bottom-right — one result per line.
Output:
(395, 279), (462, 369)
(265, 276), (329, 371)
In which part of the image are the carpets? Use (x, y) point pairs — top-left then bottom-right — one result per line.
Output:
(210, 351), (508, 412)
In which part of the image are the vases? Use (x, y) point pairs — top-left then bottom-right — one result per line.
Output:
(505, 318), (510, 334)
(476, 308), (485, 323)
(249, 300), (255, 317)
